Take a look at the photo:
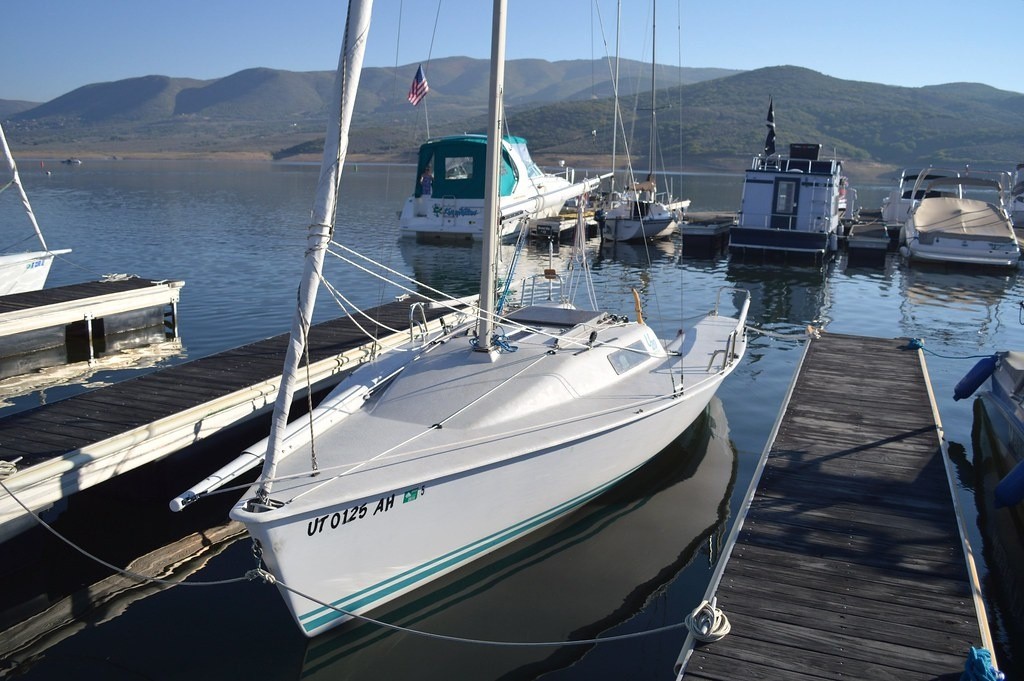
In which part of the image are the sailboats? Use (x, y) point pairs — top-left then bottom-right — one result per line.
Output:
(166, 3), (752, 641)
(298, 396), (738, 681)
(586, 0), (692, 244)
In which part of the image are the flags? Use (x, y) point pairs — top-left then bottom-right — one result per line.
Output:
(407, 64), (429, 107)
(764, 95), (776, 157)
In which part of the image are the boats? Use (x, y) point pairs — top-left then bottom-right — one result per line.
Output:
(880, 163), (1024, 266)
(0, 122), (72, 302)
(728, 143), (863, 264)
(528, 188), (619, 247)
(397, 130), (613, 243)
(679, 209), (741, 239)
(953, 344), (1023, 605)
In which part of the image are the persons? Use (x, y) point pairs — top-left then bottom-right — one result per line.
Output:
(421, 167), (433, 198)
